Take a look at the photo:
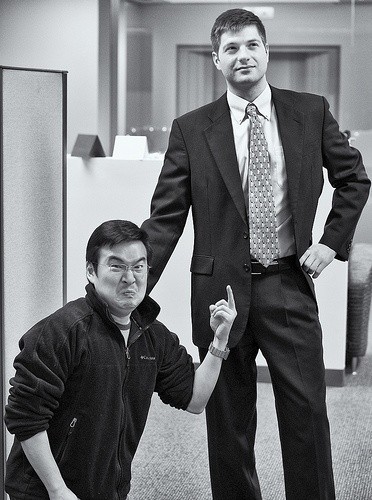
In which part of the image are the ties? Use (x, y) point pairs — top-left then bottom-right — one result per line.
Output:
(245, 104), (279, 267)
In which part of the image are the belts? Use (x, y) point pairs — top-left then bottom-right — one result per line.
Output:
(251, 254), (298, 275)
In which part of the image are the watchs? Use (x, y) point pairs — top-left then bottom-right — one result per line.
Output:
(208, 342), (230, 360)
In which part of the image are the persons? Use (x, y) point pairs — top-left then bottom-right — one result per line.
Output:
(4, 220), (238, 500)
(139, 9), (372, 500)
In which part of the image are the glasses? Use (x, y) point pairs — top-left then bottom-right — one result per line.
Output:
(92, 260), (153, 274)
